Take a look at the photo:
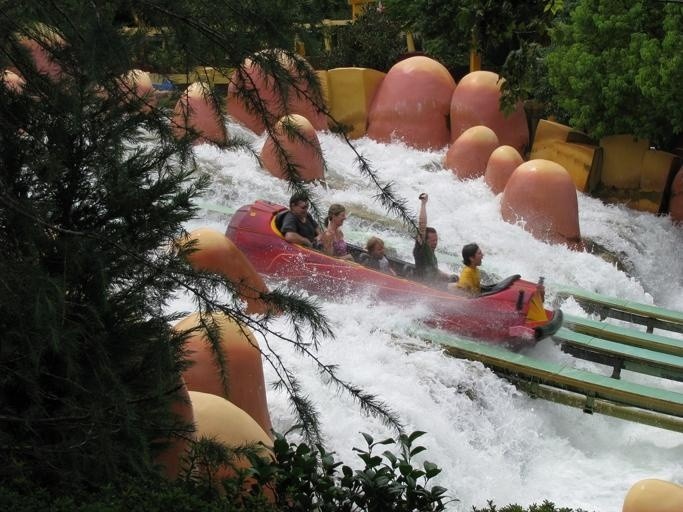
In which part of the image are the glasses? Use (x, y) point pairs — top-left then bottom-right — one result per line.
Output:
(296, 204), (309, 210)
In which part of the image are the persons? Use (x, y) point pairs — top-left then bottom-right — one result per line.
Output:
(320, 204), (354, 261)
(456, 242), (485, 296)
(411, 192), (458, 295)
(361, 237), (397, 278)
(280, 191), (322, 251)
